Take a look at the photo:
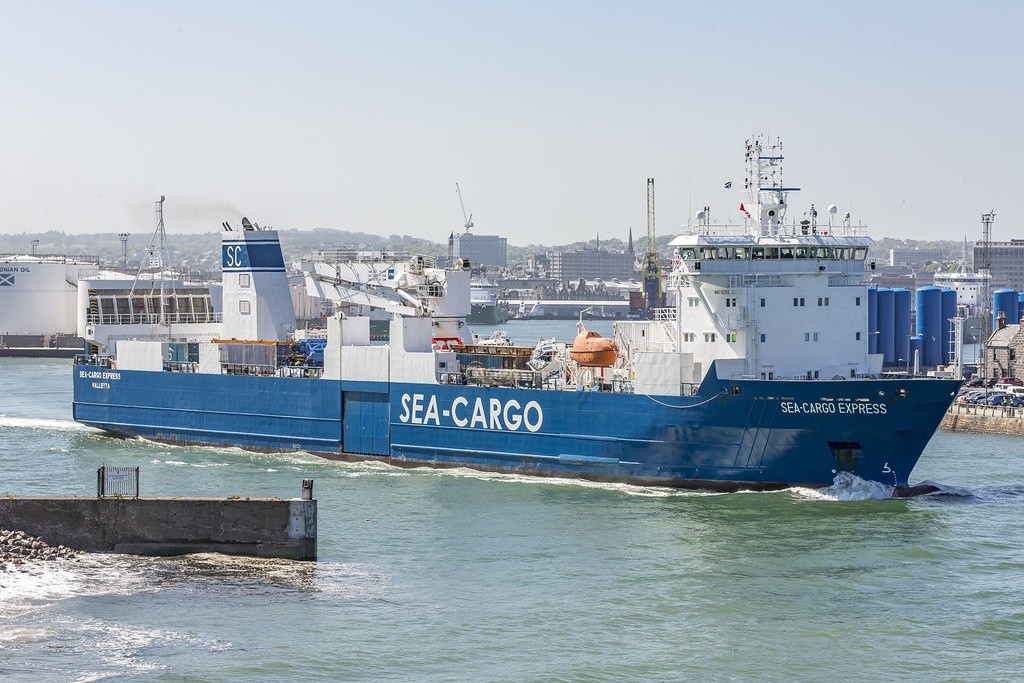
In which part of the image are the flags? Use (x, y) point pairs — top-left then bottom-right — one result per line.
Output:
(725, 182), (732, 188)
(740, 203), (751, 218)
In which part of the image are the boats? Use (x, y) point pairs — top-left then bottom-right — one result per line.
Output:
(586, 310), (616, 320)
(465, 278), (508, 326)
(478, 330), (514, 346)
(570, 330), (619, 367)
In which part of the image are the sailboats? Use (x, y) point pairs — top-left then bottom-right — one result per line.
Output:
(70, 136), (967, 497)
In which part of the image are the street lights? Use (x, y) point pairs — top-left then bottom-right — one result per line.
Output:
(973, 335), (977, 364)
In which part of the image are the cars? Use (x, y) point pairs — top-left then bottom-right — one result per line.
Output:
(956, 376), (1024, 408)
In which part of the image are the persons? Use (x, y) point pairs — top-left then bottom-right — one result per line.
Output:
(784, 250), (794, 258)
(1003, 397), (1007, 412)
(1009, 396), (1013, 406)
(799, 251), (805, 258)
